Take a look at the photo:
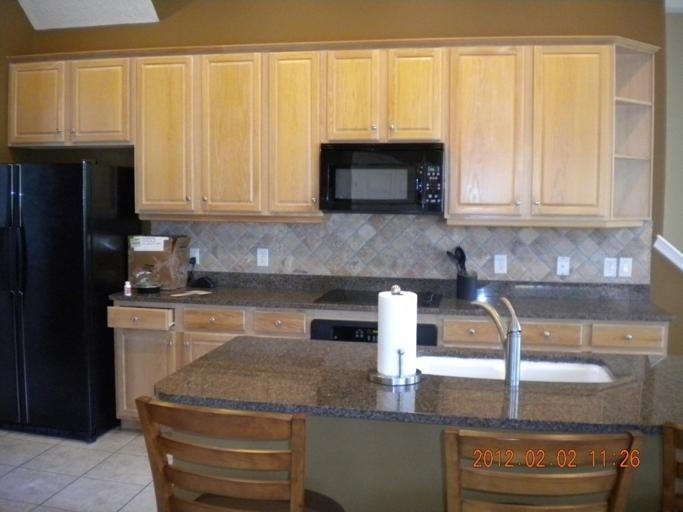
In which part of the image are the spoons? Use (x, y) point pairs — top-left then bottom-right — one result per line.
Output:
(445, 251), (460, 273)
(456, 247), (468, 272)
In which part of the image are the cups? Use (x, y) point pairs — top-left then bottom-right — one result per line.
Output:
(456, 270), (477, 301)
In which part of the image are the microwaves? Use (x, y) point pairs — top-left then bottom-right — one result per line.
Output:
(317, 142), (442, 217)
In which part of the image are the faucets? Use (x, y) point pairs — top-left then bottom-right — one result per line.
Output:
(471, 296), (522, 420)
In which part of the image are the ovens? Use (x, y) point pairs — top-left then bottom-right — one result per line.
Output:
(310, 318), (439, 346)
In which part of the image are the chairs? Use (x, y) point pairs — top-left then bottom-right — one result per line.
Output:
(658, 419), (683, 511)
(135, 396), (346, 512)
(440, 430), (647, 511)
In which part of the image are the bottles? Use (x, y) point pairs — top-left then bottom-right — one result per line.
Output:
(123, 281), (131, 297)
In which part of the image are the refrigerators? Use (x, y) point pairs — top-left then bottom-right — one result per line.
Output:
(1, 162), (141, 443)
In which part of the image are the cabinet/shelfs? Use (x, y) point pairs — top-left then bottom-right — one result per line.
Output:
(445, 49), (608, 221)
(316, 49), (443, 143)
(5, 56), (129, 145)
(264, 50), (316, 215)
(610, 43), (655, 219)
(130, 50), (261, 219)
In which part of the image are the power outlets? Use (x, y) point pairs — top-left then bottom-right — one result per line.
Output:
(189, 248), (200, 265)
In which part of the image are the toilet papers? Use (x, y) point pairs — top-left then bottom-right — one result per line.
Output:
(374, 290), (422, 377)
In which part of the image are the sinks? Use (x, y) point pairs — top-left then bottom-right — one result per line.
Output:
(416, 355), (616, 384)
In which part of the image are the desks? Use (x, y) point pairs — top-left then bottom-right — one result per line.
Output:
(152, 334), (683, 511)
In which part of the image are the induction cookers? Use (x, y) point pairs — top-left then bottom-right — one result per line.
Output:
(313, 289), (441, 308)
